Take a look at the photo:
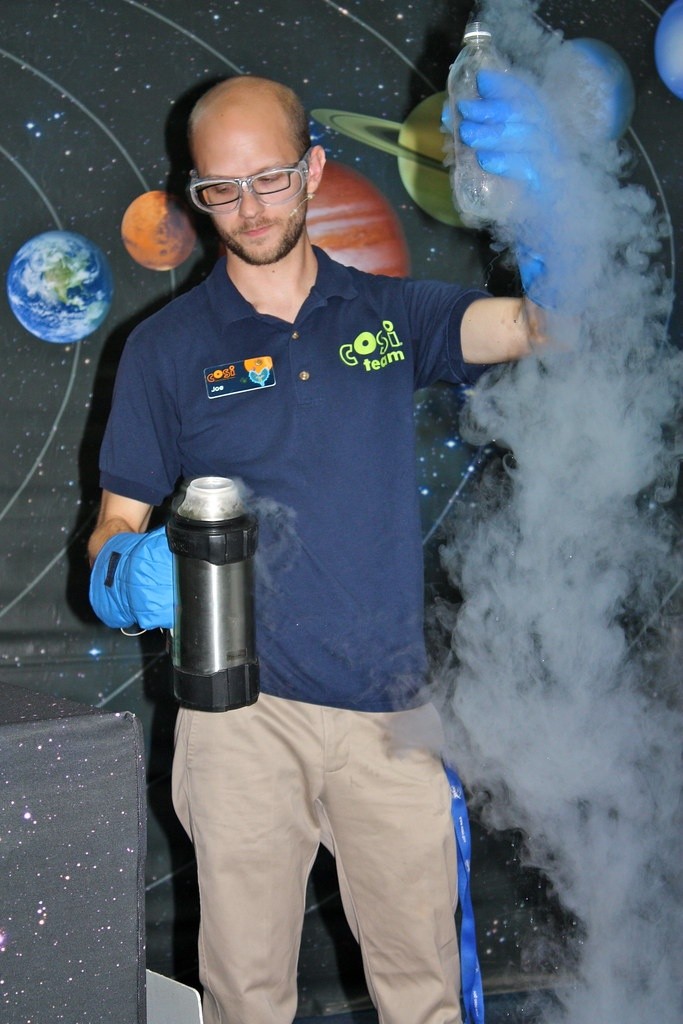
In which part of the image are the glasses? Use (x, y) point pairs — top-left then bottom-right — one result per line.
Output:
(197, 147), (309, 207)
(189, 146), (314, 215)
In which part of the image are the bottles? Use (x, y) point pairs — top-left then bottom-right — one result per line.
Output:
(448, 22), (517, 219)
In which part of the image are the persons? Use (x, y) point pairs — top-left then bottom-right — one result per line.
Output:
(85, 69), (583, 1024)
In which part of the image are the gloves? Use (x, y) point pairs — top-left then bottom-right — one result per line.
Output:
(441, 70), (602, 315)
(88, 526), (174, 631)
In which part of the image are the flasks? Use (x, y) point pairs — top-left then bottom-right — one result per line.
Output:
(160, 473), (263, 713)
